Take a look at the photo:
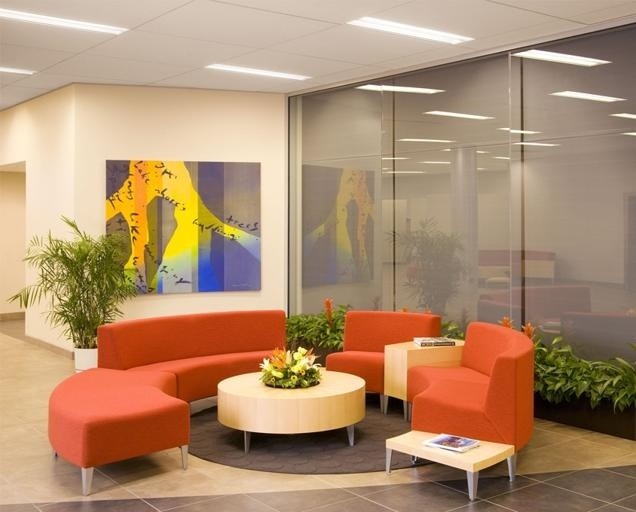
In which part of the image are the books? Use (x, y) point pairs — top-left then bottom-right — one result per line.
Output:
(543, 319), (561, 331)
(424, 432), (481, 454)
(413, 335), (456, 347)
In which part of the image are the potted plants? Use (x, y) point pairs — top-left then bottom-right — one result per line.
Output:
(4, 212), (142, 374)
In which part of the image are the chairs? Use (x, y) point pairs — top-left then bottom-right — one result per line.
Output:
(325, 312), (535, 471)
(476, 287), (635, 369)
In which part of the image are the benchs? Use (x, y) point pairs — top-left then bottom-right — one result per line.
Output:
(46, 368), (191, 497)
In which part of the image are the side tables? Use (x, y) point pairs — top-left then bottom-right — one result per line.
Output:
(384, 431), (515, 502)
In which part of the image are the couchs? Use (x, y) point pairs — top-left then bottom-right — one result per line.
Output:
(96, 309), (285, 417)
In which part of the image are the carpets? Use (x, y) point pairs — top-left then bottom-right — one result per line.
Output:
(179, 394), (437, 474)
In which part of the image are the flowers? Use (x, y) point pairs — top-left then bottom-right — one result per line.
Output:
(255, 344), (322, 390)
